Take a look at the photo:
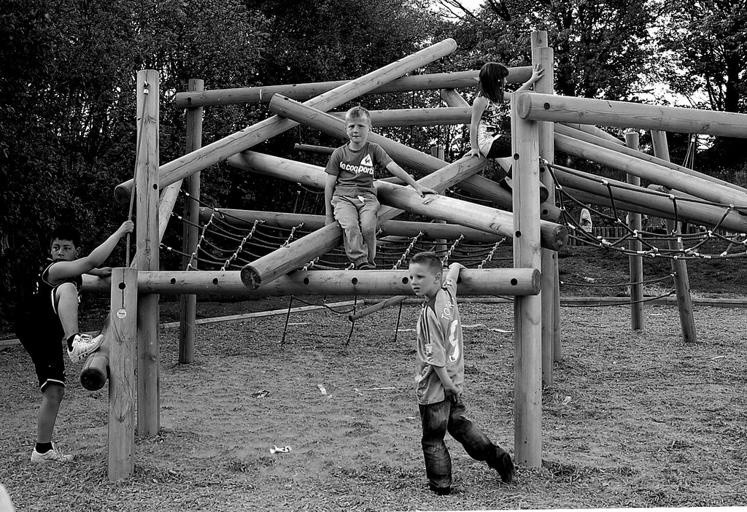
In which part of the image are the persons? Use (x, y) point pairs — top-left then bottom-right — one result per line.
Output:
(409, 252), (515, 495)
(463, 62), (545, 193)
(324, 106), (438, 268)
(17, 218), (136, 464)
(579, 202), (592, 235)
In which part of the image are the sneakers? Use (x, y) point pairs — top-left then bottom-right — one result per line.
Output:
(67, 333), (105, 363)
(429, 484), (450, 494)
(357, 262), (376, 269)
(499, 178), (512, 193)
(496, 453), (514, 482)
(31, 441), (73, 461)
